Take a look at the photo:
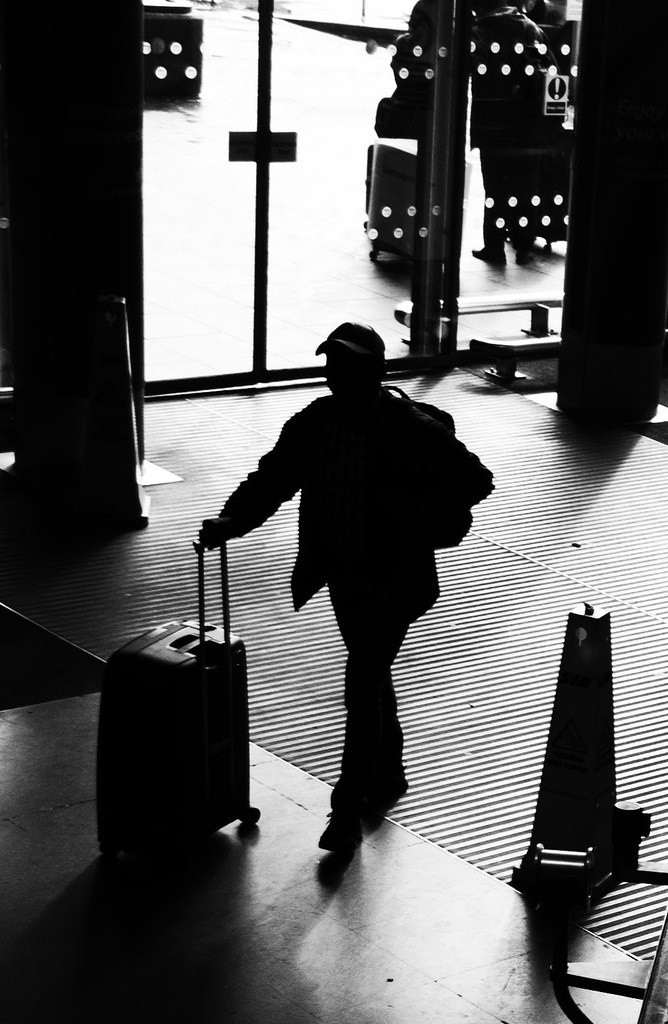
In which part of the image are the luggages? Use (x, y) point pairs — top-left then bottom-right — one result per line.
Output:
(95, 543), (262, 873)
(364, 137), (418, 274)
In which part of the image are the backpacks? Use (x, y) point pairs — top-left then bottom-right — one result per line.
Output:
(383, 384), (496, 550)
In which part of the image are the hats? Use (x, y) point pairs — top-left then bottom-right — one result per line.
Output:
(314, 321), (385, 365)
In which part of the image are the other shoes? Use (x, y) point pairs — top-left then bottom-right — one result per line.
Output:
(516, 248), (535, 264)
(319, 809), (365, 852)
(473, 249), (506, 266)
(362, 778), (408, 813)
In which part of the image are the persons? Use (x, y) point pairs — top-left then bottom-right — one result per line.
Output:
(373, 0), (568, 266)
(200, 322), (471, 852)
